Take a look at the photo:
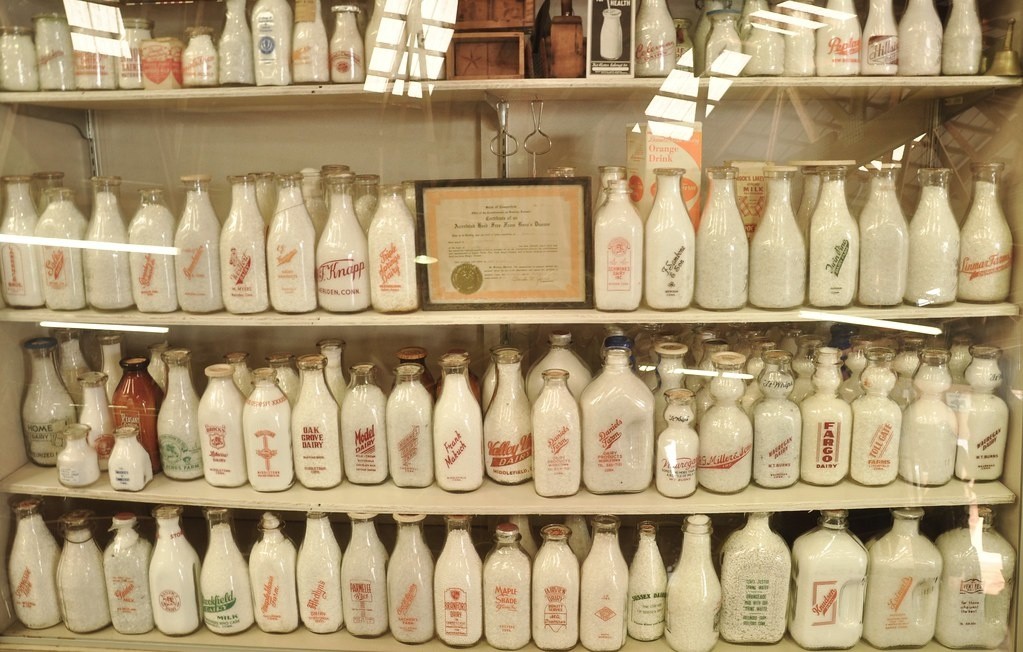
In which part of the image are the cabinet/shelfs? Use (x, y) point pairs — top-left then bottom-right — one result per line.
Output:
(0, 0), (1021, 652)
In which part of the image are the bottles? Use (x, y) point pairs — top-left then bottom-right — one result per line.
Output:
(1, 165), (419, 313)
(30, 12), (75, 90)
(149, 505), (203, 637)
(249, 509), (299, 633)
(531, 514), (592, 651)
(340, 513), (389, 637)
(482, 515), (539, 650)
(386, 514), (436, 644)
(116, 17), (154, 88)
(529, 330), (594, 495)
(628, 521), (667, 642)
(182, 26), (217, 86)
(862, 507), (944, 649)
(663, 514), (722, 652)
(599, 8), (623, 59)
(719, 511), (791, 644)
(791, 508), (869, 651)
(217, 0), (255, 86)
(103, 512), (155, 634)
(579, 324), (1009, 499)
(329, 4), (366, 84)
(291, 0), (331, 84)
(72, 25), (120, 91)
(200, 507), (255, 635)
(934, 504), (1017, 650)
(296, 512), (344, 634)
(632, 0), (982, 77)
(433, 515), (482, 647)
(55, 509), (111, 633)
(581, 514), (629, 651)
(24, 329), (534, 490)
(0, 26), (40, 91)
(251, 0), (293, 86)
(9, 494), (62, 628)
(544, 162), (1011, 312)
